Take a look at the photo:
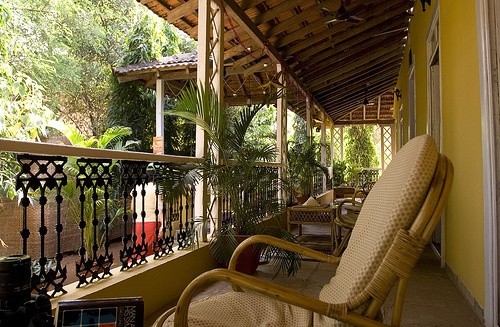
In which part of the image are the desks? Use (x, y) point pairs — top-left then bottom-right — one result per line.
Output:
(287, 206), (341, 253)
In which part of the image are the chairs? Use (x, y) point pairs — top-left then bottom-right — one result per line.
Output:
(152, 134), (453, 327)
(332, 182), (375, 256)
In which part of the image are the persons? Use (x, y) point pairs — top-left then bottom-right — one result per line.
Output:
(130, 169), (167, 260)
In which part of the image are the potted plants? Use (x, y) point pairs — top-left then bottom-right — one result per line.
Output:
(279, 136), (330, 204)
(60, 125), (143, 278)
(151, 79), (303, 281)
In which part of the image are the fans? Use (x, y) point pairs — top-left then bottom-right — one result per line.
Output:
(321, 0), (367, 26)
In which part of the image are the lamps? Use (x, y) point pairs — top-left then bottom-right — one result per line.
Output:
(394, 88), (401, 101)
